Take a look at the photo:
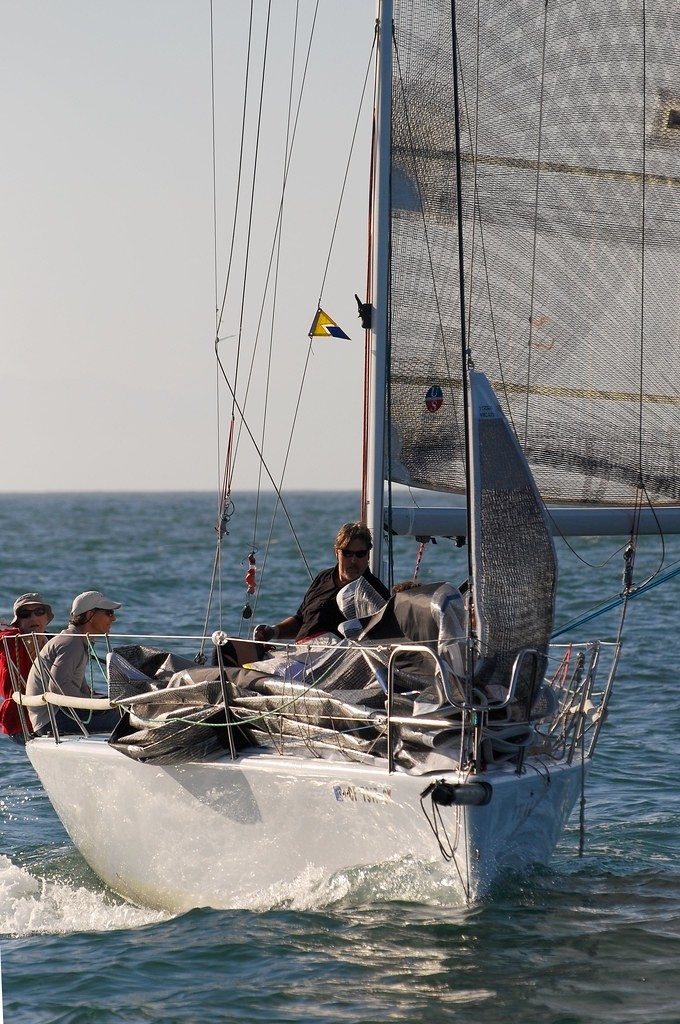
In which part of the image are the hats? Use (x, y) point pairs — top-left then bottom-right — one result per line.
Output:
(72, 591), (121, 616)
(10, 593), (54, 628)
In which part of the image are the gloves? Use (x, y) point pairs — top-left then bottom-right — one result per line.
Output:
(253, 624), (280, 641)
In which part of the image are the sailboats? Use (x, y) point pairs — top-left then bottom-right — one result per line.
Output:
(0, 0), (680, 920)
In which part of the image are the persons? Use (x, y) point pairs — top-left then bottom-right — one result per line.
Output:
(0, 593), (57, 746)
(26, 590), (131, 737)
(211, 520), (407, 669)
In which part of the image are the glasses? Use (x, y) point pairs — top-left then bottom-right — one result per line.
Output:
(93, 610), (114, 617)
(17, 607), (46, 618)
(337, 548), (368, 558)
